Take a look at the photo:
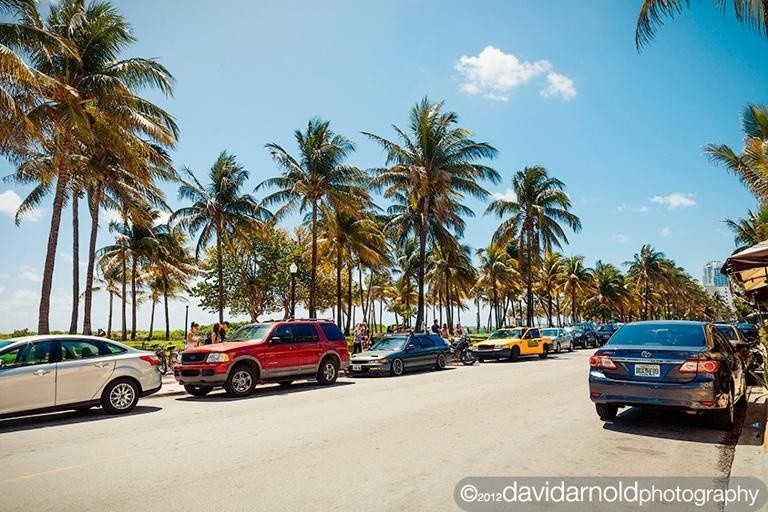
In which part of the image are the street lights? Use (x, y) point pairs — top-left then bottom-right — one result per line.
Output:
(289, 263), (298, 319)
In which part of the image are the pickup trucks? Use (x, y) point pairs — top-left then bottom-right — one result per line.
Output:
(472, 326), (552, 362)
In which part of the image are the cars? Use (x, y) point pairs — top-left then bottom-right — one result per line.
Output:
(590, 320), (749, 429)
(712, 323), (758, 372)
(346, 332), (450, 377)
(2, 334), (162, 423)
(596, 322), (623, 344)
(540, 329), (575, 353)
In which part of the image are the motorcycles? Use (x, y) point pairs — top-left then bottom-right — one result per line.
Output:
(452, 335), (476, 366)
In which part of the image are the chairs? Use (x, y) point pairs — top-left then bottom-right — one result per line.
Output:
(33, 347), (49, 360)
(64, 347), (93, 358)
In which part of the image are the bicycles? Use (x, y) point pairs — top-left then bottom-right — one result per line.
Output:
(163, 343), (180, 370)
(140, 337), (167, 375)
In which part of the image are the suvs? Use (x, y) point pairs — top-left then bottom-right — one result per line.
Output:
(565, 321), (599, 349)
(173, 319), (350, 397)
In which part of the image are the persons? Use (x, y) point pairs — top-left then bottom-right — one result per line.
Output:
(432, 318), (468, 338)
(181, 318), (229, 349)
(349, 321), (374, 353)
